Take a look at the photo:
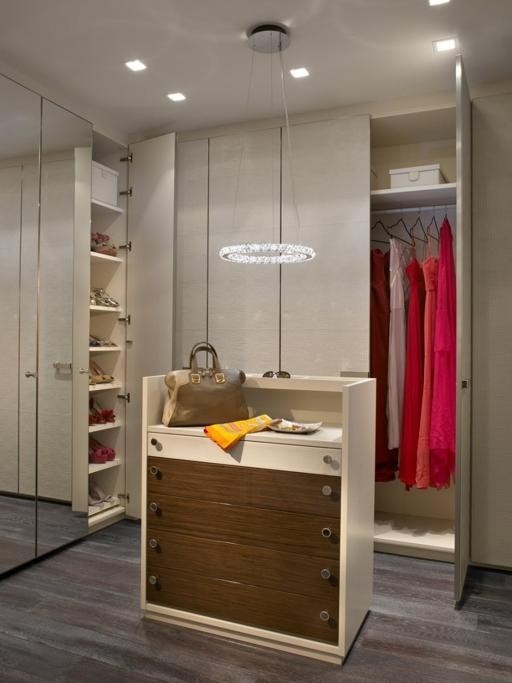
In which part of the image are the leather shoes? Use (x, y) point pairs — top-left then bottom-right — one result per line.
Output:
(89, 232), (120, 508)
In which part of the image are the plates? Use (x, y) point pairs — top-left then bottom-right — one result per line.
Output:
(265, 418), (323, 435)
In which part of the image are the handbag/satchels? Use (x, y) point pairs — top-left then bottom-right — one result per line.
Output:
(158, 341), (250, 429)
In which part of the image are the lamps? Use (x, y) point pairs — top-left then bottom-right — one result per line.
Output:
(220, 20), (316, 266)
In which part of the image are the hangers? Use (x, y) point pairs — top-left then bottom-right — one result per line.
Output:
(368, 204), (455, 256)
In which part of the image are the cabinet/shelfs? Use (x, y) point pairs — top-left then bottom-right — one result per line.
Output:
(179, 52), (477, 604)
(79, 132), (178, 522)
(137, 369), (379, 667)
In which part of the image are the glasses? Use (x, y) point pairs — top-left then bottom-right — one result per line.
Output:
(262, 370), (291, 379)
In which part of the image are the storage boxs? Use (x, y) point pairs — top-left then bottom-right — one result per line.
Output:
(386, 162), (448, 192)
(87, 155), (121, 208)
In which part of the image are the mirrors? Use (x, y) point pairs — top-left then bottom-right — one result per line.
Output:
(1, 74), (94, 575)
